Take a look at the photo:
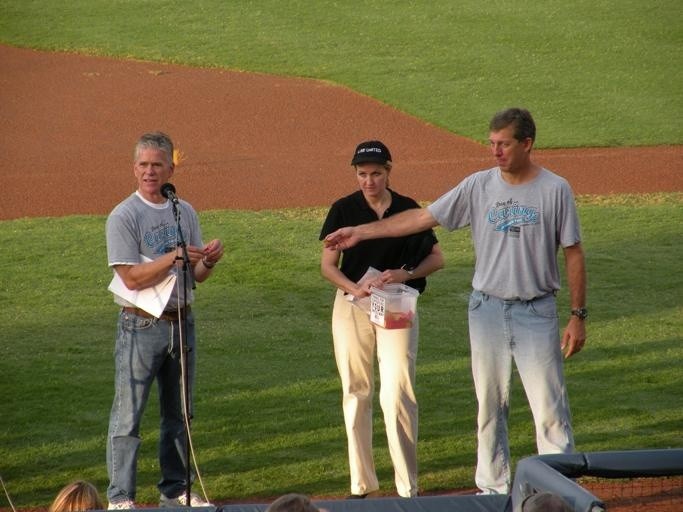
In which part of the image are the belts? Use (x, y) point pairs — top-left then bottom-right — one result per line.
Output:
(123, 306), (191, 320)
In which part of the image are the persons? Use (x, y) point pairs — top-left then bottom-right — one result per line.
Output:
(324, 107), (587, 495)
(265, 493), (328, 512)
(516, 491), (576, 512)
(105, 130), (225, 510)
(317, 140), (445, 500)
(49, 480), (103, 512)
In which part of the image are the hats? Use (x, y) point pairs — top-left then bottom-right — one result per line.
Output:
(352, 141), (391, 164)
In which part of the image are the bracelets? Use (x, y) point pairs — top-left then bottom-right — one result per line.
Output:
(201, 254), (216, 269)
(408, 266), (416, 280)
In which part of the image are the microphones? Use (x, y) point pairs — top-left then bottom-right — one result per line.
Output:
(160, 183), (179, 204)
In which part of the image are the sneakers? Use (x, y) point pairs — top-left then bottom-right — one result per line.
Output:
(107, 502), (133, 510)
(159, 491), (214, 507)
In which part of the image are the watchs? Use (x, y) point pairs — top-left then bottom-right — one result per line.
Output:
(571, 307), (589, 320)
